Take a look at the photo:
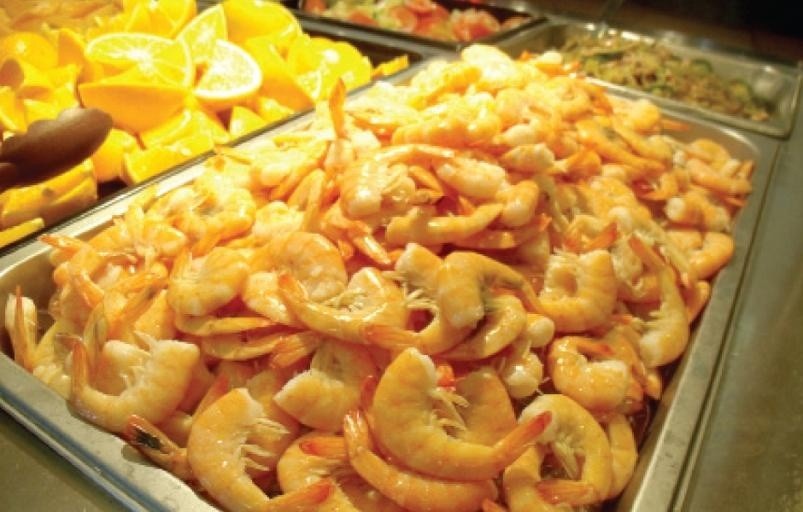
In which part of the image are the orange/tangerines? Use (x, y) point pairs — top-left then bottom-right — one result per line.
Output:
(1, 0), (410, 244)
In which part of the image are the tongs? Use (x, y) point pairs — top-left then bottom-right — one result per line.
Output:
(1, 106), (113, 193)
